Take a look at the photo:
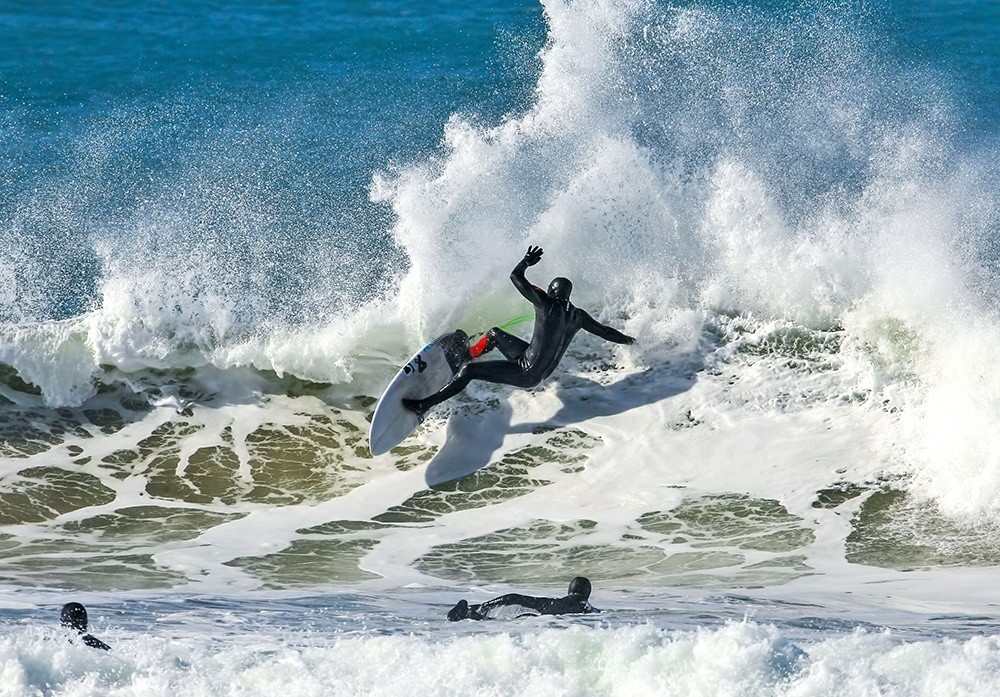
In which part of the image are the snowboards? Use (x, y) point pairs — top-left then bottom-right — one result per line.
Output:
(369, 331), (471, 456)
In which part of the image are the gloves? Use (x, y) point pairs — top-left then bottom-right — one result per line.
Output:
(524, 245), (544, 266)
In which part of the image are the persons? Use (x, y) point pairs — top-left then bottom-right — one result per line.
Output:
(60, 601), (112, 652)
(401, 246), (637, 413)
(447, 576), (600, 621)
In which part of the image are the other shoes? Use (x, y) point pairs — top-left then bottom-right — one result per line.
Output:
(447, 600), (469, 622)
(455, 334), (471, 363)
(402, 398), (428, 415)
